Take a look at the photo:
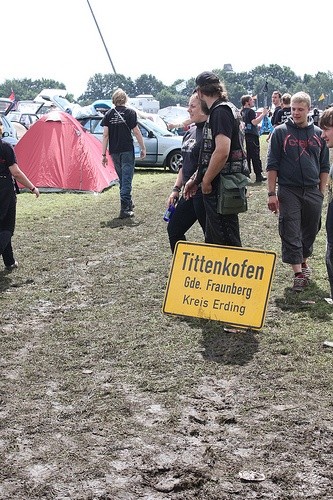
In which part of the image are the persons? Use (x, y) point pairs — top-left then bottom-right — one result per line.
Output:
(168, 118), (193, 129)
(265, 91), (331, 291)
(101, 90), (146, 217)
(270, 91), (282, 125)
(319, 106), (333, 300)
(166, 91), (208, 255)
(274, 93), (291, 126)
(0, 118), (39, 269)
(311, 109), (320, 126)
(240, 96), (267, 182)
(182, 72), (242, 247)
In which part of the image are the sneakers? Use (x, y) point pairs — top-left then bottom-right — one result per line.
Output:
(292, 274), (309, 291)
(301, 264), (312, 284)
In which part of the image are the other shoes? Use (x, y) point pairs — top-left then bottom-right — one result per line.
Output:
(6, 261), (18, 269)
(256, 175), (267, 182)
(120, 210), (134, 218)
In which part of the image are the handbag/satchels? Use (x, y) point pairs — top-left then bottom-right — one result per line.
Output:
(217, 172), (248, 215)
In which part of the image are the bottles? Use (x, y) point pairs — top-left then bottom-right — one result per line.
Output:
(163, 203), (175, 221)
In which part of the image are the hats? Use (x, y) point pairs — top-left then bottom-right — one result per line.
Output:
(192, 71), (219, 93)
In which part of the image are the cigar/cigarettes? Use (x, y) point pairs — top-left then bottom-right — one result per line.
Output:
(272, 211), (275, 214)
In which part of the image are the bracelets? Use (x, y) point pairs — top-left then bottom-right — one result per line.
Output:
(173, 187), (181, 193)
(190, 174), (195, 180)
(102, 154), (106, 156)
(268, 192), (276, 196)
(32, 188), (35, 191)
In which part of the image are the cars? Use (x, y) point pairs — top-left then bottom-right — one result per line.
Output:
(75, 115), (184, 174)
(0, 87), (192, 148)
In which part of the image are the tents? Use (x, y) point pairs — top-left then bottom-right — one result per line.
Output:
(14, 110), (119, 194)
